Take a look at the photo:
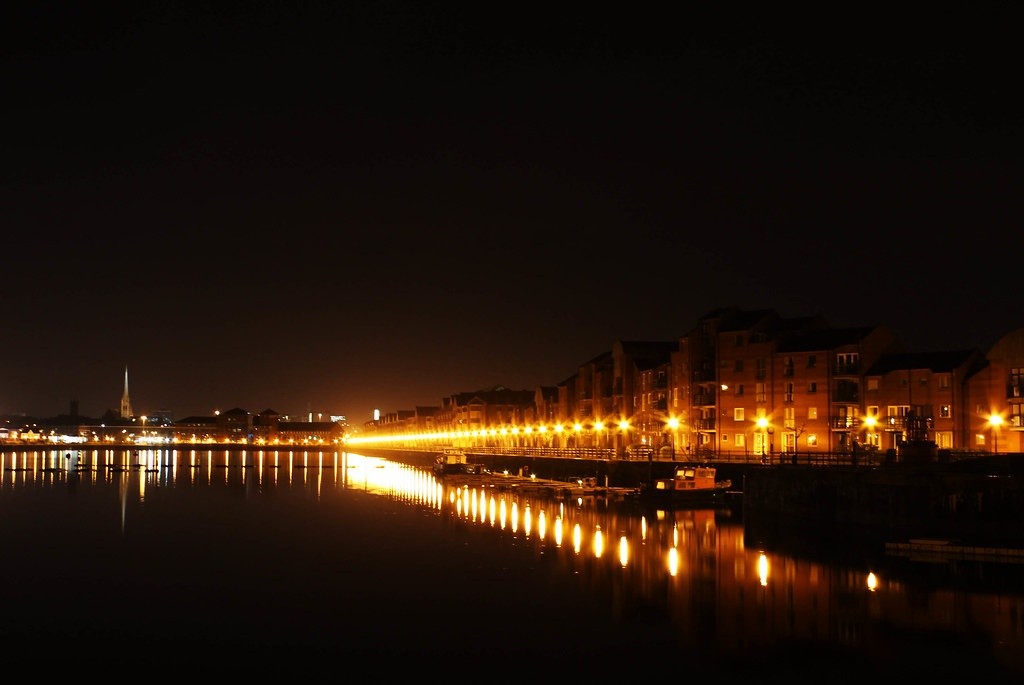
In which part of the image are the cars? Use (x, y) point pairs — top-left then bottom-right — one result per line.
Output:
(626, 444), (653, 457)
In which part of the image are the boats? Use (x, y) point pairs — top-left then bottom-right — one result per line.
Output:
(432, 447), (733, 503)
